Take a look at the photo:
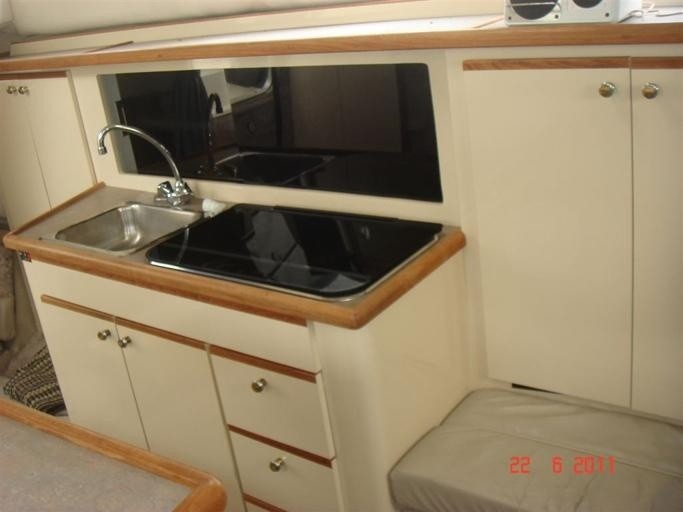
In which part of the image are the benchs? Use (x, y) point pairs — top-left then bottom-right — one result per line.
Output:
(388, 389), (683, 512)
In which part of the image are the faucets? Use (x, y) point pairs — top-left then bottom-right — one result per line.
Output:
(203, 91), (222, 166)
(97, 124), (192, 206)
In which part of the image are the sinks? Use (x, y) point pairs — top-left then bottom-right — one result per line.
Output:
(199, 152), (333, 185)
(40, 199), (203, 259)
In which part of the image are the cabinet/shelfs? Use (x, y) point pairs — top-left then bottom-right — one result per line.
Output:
(39, 295), (246, 511)
(209, 346), (344, 512)
(444, 41), (683, 423)
(0, 71), (96, 234)
